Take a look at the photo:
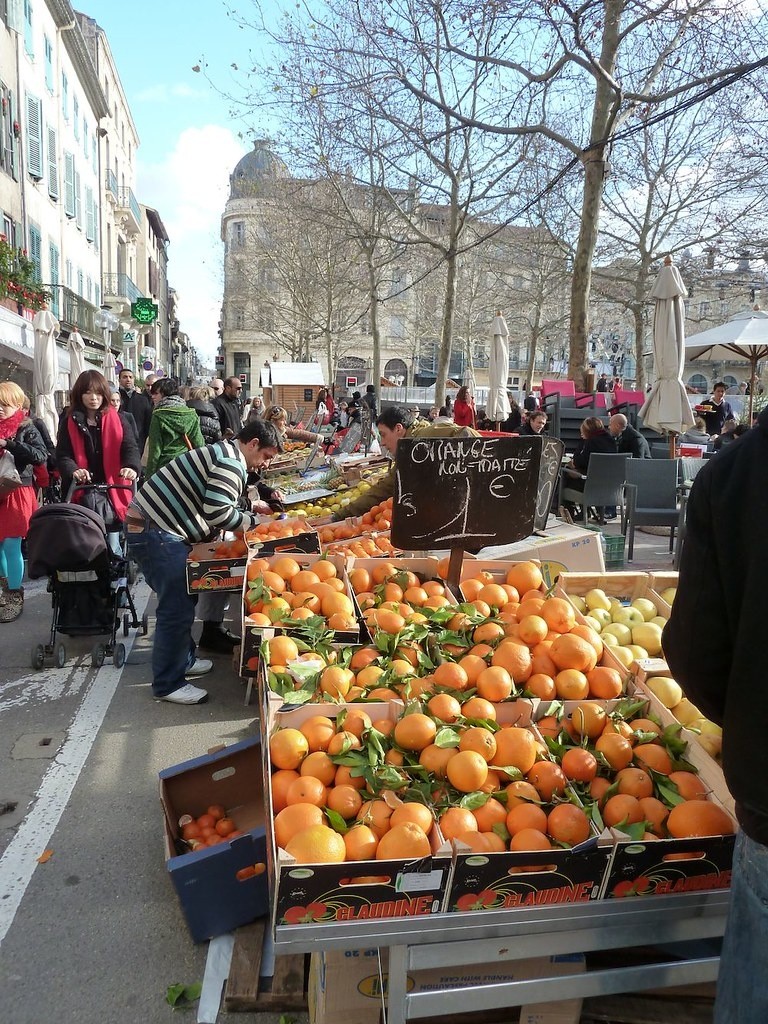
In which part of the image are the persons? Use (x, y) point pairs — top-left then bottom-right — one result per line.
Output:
(660, 396), (768, 1024)
(315, 405), (488, 555)
(0, 370), (381, 706)
(401, 368), (766, 527)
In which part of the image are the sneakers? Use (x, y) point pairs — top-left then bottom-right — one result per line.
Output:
(185, 657), (213, 675)
(153, 683), (209, 704)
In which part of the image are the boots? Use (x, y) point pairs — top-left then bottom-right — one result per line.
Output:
(0, 580), (24, 623)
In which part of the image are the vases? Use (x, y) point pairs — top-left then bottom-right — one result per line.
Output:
(0, 294), (20, 314)
(22, 304), (36, 319)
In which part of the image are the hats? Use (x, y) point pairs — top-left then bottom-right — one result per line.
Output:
(411, 406), (421, 412)
(335, 410), (340, 415)
(367, 385), (375, 393)
(724, 420), (739, 430)
(353, 391), (361, 399)
(109, 386), (120, 397)
(348, 401), (361, 407)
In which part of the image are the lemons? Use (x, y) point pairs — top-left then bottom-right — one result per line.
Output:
(267, 465), (395, 517)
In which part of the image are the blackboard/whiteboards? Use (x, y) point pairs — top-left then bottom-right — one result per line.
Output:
(360, 409), (373, 446)
(513, 435), (565, 531)
(316, 415), (323, 433)
(304, 415), (313, 430)
(294, 406), (306, 423)
(307, 419), (314, 431)
(286, 411), (292, 426)
(303, 438), (320, 475)
(340, 422), (360, 454)
(281, 489), (337, 505)
(390, 438), (543, 550)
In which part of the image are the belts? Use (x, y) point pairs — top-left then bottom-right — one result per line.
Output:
(125, 515), (160, 529)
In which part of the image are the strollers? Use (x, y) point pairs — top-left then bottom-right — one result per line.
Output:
(25, 472), (149, 671)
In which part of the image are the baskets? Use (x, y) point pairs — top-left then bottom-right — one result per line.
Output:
(334, 428), (361, 453)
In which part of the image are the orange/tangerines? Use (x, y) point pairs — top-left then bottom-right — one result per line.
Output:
(184, 806), (265, 879)
(270, 694), (735, 883)
(190, 497), (393, 570)
(247, 557), (623, 701)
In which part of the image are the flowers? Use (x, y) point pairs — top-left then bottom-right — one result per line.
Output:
(0, 233), (48, 308)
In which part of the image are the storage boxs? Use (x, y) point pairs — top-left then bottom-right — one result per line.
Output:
(158, 456), (741, 1024)
(680, 443), (707, 460)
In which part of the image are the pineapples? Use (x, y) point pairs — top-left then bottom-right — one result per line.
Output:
(299, 481), (318, 491)
(329, 476), (345, 488)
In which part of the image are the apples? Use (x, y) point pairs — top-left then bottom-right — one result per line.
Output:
(567, 587), (723, 766)
(284, 441), (306, 451)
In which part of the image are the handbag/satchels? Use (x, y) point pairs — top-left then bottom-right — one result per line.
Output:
(0, 449), (23, 494)
(368, 438), (382, 456)
(79, 482), (119, 525)
(314, 414), (331, 425)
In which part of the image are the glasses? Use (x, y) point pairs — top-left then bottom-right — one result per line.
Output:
(232, 387), (243, 390)
(268, 407), (282, 421)
(210, 386), (219, 391)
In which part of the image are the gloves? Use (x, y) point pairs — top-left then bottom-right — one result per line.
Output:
(324, 437), (335, 446)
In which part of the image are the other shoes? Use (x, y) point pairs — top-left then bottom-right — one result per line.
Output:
(604, 514), (617, 520)
(575, 511), (591, 521)
(592, 516), (607, 525)
(198, 635), (233, 655)
(224, 631), (242, 645)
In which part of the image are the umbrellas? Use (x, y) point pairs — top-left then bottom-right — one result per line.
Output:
(66, 328), (87, 406)
(677, 304), (768, 432)
(461, 366), (478, 418)
(637, 253), (695, 460)
(103, 349), (118, 391)
(32, 302), (61, 448)
(485, 310), (512, 434)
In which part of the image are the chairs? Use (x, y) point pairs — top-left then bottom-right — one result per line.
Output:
(521, 378), (709, 570)
